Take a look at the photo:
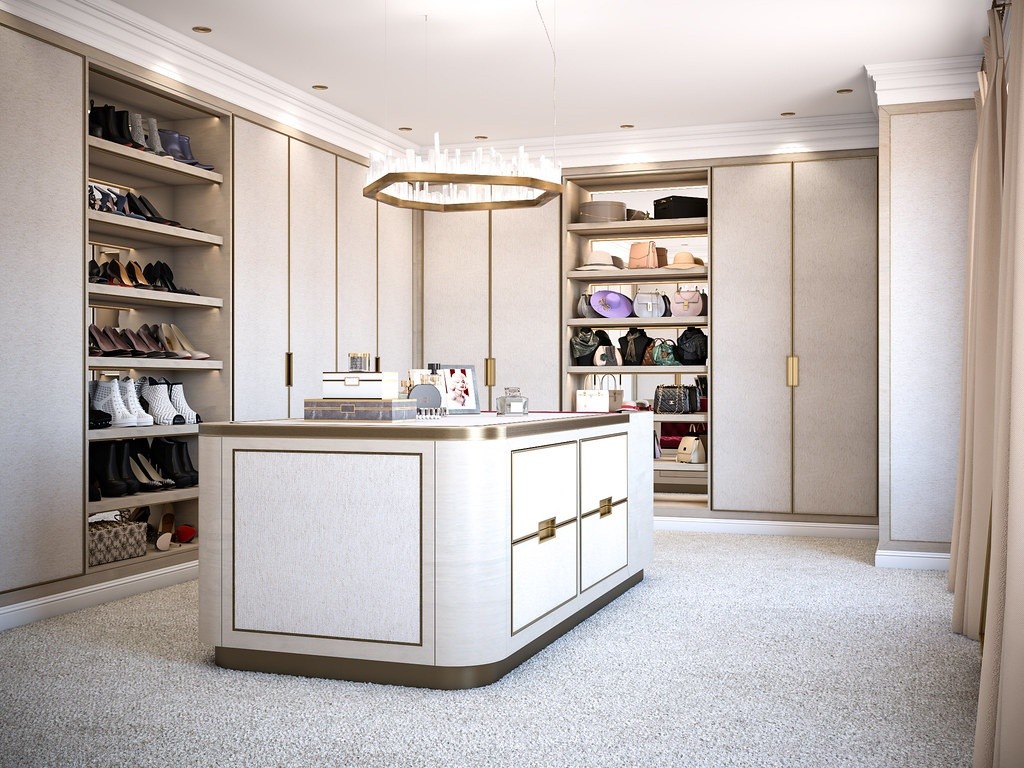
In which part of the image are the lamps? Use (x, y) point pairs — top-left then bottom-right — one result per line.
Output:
(363, 0), (564, 212)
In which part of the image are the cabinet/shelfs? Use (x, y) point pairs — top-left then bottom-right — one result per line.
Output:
(0, 9), (879, 595)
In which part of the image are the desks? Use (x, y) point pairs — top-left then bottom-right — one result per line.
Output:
(198, 411), (654, 690)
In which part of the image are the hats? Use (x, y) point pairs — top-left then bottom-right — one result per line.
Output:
(575, 251), (621, 271)
(661, 294), (672, 316)
(590, 291), (632, 319)
(693, 257), (705, 267)
(664, 252), (702, 269)
(612, 255), (629, 271)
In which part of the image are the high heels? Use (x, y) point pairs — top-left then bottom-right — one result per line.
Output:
(87, 100), (215, 552)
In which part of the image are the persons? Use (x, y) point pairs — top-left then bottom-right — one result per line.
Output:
(446, 373), (475, 409)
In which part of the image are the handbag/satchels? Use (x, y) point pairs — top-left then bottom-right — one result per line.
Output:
(696, 293), (709, 316)
(632, 292), (665, 318)
(628, 241), (658, 268)
(577, 293), (601, 317)
(669, 291), (702, 316)
(575, 336), (707, 463)
(655, 247), (667, 268)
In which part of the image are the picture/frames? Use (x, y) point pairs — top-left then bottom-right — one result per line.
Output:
(439, 365), (481, 414)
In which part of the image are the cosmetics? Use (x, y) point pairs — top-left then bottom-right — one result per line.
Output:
(496, 387), (529, 416)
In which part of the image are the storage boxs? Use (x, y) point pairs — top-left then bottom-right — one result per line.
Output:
(577, 390), (623, 413)
(322, 371), (398, 399)
(88, 520), (147, 566)
(304, 399), (417, 422)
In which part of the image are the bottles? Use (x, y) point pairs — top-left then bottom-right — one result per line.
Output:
(496, 387), (529, 417)
